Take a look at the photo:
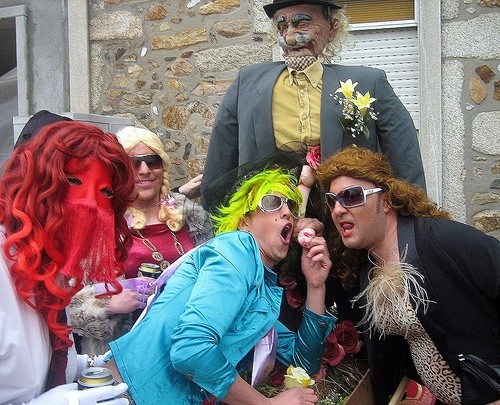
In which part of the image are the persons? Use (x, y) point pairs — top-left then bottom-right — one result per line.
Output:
(200, 0), (427, 251)
(314, 143), (500, 405)
(95, 170), (338, 405)
(0, 121), (130, 405)
(68, 126), (217, 357)
(170, 171), (204, 197)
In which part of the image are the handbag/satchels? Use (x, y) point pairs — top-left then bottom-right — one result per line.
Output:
(455, 352), (500, 405)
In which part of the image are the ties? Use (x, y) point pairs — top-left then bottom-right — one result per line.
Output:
(44, 308), (68, 392)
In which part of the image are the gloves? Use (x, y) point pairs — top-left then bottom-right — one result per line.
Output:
(94, 349), (112, 366)
(26, 383), (129, 405)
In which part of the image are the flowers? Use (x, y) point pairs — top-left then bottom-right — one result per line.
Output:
(278, 272), (301, 308)
(284, 321), (361, 389)
(331, 79), (382, 142)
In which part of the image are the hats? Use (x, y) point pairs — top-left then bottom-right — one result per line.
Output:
(263, 0), (342, 20)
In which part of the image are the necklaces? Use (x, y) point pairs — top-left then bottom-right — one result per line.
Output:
(135, 217), (186, 275)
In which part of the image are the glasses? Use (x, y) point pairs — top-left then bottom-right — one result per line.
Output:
(129, 153), (164, 169)
(324, 185), (383, 212)
(257, 194), (300, 220)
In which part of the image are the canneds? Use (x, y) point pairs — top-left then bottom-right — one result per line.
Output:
(77, 367), (116, 403)
(137, 263), (162, 280)
